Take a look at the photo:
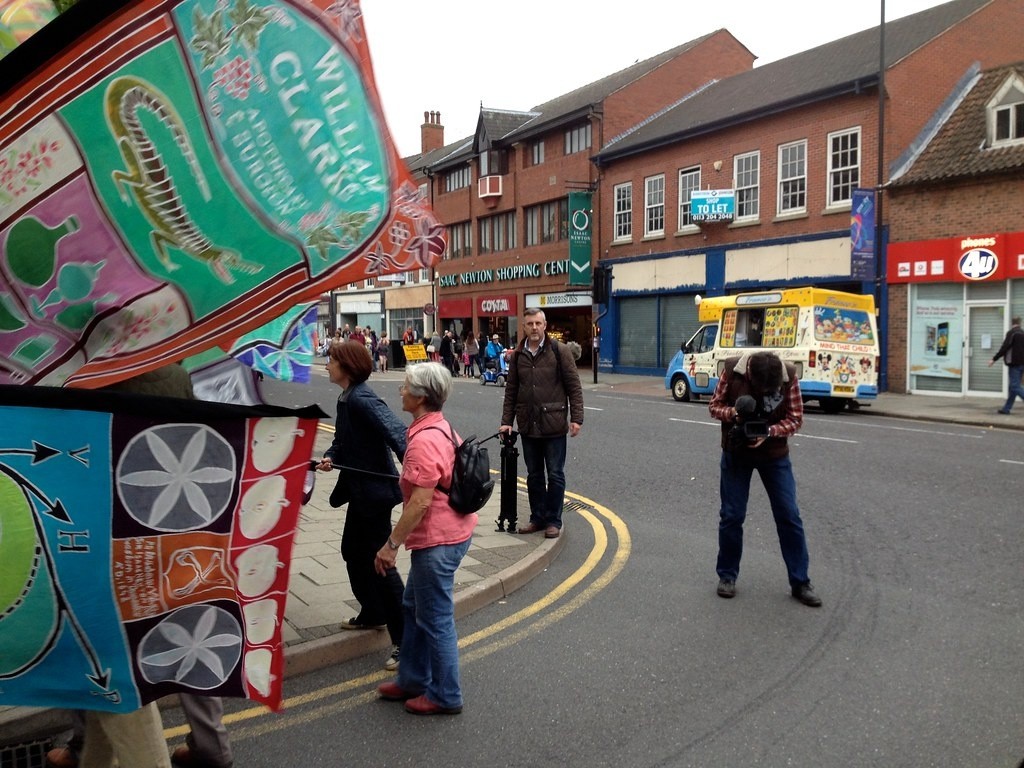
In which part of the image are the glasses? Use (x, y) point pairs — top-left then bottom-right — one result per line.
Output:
(398, 384), (408, 391)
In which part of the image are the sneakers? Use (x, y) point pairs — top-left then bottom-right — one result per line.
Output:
(717, 579), (736, 597)
(792, 582), (822, 607)
(405, 695), (462, 714)
(378, 681), (406, 700)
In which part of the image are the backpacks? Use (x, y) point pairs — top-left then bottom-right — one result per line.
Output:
(423, 420), (494, 515)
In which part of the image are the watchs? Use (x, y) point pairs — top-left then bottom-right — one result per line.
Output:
(388, 536), (399, 549)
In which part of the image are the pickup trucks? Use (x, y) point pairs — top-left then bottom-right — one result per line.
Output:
(664, 287), (880, 414)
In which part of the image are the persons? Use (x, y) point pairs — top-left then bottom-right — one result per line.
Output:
(499, 308), (585, 538)
(987, 316), (1024, 414)
(322, 323), (390, 371)
(48, 359), (234, 768)
(708, 350), (822, 607)
(374, 362), (478, 715)
(403, 325), (503, 379)
(313, 341), (408, 671)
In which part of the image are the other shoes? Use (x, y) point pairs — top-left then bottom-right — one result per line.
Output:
(998, 409), (1010, 414)
(385, 645), (402, 670)
(342, 615), (387, 630)
(466, 375), (469, 377)
(472, 376), (475, 379)
(463, 374), (465, 377)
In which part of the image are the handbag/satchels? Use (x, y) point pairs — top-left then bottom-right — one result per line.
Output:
(426, 345), (436, 352)
(454, 359), (460, 371)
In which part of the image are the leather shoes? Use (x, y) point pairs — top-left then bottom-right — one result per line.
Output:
(519, 522), (539, 534)
(545, 525), (559, 538)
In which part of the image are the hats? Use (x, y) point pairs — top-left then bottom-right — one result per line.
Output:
(492, 334), (500, 339)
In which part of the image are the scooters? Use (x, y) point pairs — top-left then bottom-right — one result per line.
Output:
(480, 348), (510, 386)
